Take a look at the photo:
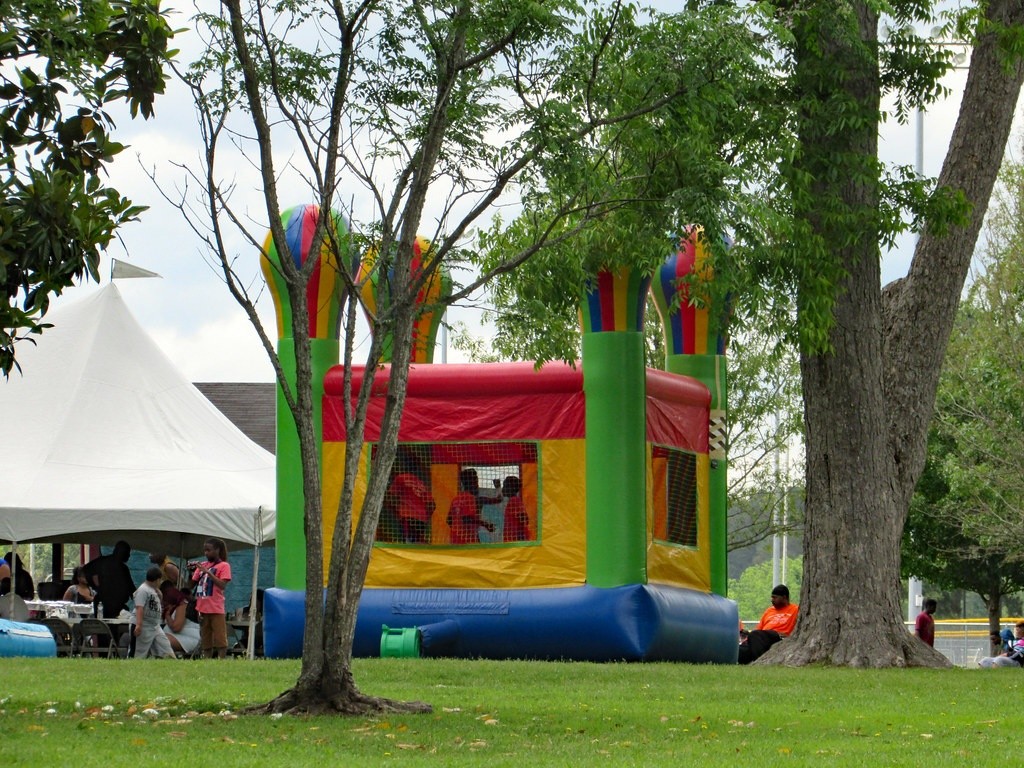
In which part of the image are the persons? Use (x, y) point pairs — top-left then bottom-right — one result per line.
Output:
(0, 537), (266, 658)
(978, 622), (1024, 669)
(738, 585), (798, 664)
(501, 475), (530, 541)
(384, 461), (436, 543)
(446, 468), (503, 545)
(915, 599), (937, 648)
(739, 629), (749, 645)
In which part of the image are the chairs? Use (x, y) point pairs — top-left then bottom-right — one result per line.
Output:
(37, 618), (120, 659)
(173, 621), (247, 660)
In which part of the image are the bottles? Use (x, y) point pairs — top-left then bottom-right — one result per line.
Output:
(97, 602), (103, 620)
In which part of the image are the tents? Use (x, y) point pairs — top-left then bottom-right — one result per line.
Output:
(0, 260), (278, 657)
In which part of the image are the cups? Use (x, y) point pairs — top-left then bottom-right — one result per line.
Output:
(235, 608), (243, 622)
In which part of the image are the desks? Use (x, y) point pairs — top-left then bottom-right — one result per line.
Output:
(61, 617), (136, 658)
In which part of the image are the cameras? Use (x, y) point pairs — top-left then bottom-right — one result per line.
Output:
(189, 561), (199, 571)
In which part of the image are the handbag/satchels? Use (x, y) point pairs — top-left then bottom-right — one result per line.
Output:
(185, 598), (199, 624)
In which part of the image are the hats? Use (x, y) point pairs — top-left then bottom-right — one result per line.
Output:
(772, 585), (790, 599)
